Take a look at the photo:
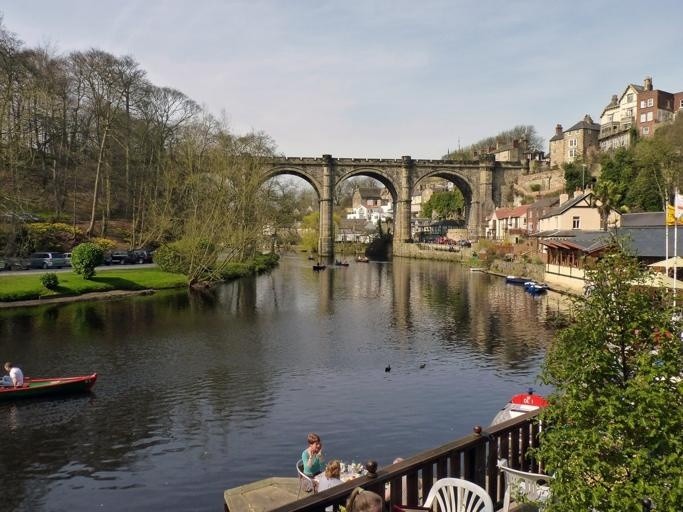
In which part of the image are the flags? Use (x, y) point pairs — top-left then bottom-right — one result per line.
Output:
(675, 193), (683, 223)
(666, 203), (683, 225)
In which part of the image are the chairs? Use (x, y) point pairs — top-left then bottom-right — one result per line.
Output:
(296, 459), (315, 501)
(499, 458), (555, 511)
(422, 478), (494, 512)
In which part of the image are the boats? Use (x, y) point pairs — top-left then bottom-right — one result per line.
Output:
(506, 276), (549, 294)
(307, 256), (368, 271)
(1, 372), (97, 401)
(490, 388), (553, 471)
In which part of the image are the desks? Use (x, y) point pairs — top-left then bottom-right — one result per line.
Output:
(314, 471), (360, 486)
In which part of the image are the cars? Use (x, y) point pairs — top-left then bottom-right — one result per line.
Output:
(0, 249), (152, 270)
(1, 212), (41, 224)
(420, 237), (473, 248)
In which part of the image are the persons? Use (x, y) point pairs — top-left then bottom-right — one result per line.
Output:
(317, 459), (344, 512)
(345, 486), (382, 512)
(302, 431), (322, 479)
(4, 361), (23, 389)
